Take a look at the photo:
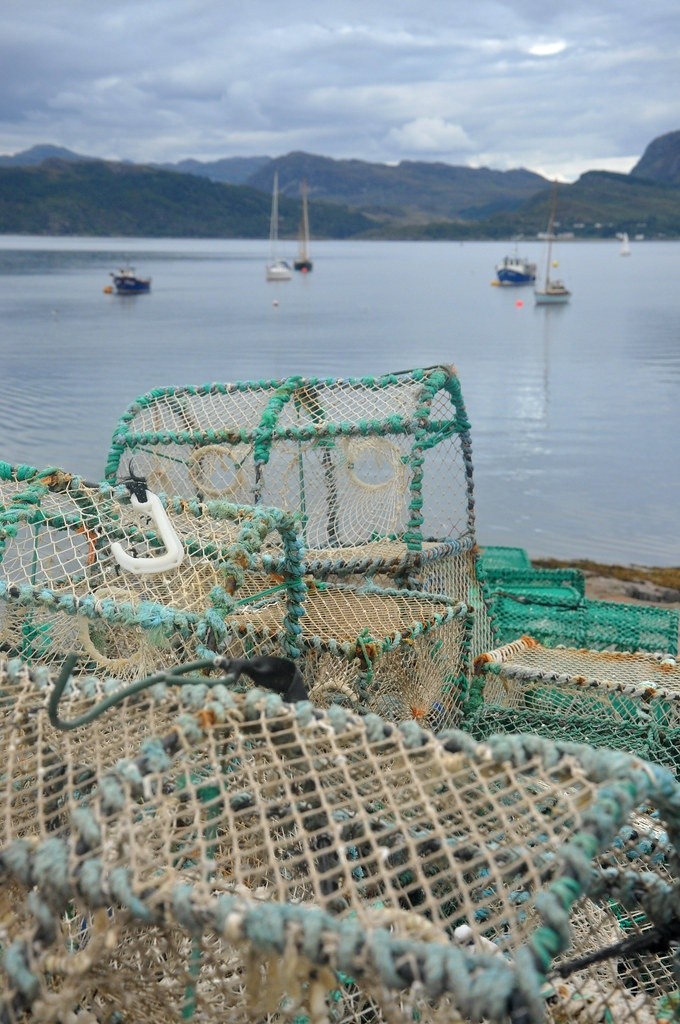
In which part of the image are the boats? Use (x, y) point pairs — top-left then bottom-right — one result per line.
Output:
(497, 258), (538, 287)
(104, 267), (153, 296)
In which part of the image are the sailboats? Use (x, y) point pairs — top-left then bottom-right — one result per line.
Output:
(532, 195), (574, 306)
(293, 184), (319, 272)
(268, 170), (294, 281)
